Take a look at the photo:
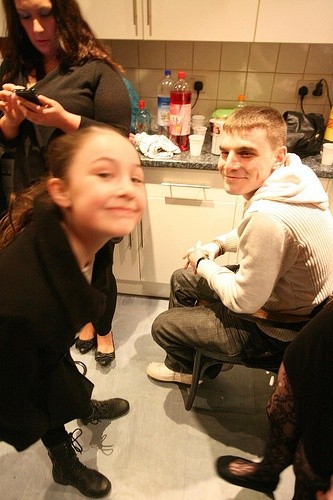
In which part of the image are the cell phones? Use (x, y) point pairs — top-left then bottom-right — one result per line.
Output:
(15, 89), (41, 107)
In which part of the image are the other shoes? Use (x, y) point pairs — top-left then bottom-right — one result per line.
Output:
(95, 331), (115, 367)
(75, 332), (97, 354)
(215, 454), (280, 493)
(146, 361), (203, 385)
(221, 363), (233, 372)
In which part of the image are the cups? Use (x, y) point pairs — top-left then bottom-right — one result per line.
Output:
(193, 126), (207, 136)
(188, 135), (205, 156)
(192, 115), (206, 126)
(321, 143), (333, 165)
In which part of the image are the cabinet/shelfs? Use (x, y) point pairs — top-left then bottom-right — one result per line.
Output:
(0, 0), (333, 45)
(113, 166), (333, 302)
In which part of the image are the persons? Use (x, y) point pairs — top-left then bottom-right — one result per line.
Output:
(0, 0), (131, 367)
(215, 293), (333, 500)
(0, 124), (145, 498)
(147, 104), (333, 389)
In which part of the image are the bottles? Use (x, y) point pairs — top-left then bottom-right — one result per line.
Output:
(235, 95), (247, 109)
(157, 69), (174, 139)
(168, 72), (192, 151)
(135, 100), (151, 136)
(319, 105), (333, 153)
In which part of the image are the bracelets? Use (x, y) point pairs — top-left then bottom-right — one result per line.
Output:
(195, 256), (210, 271)
(212, 239), (225, 256)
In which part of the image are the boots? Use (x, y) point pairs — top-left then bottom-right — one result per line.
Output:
(80, 398), (129, 426)
(45, 428), (111, 499)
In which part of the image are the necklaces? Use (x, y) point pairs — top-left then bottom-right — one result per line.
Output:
(81, 255), (94, 272)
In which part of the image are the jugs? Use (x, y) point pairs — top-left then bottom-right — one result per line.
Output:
(209, 109), (235, 156)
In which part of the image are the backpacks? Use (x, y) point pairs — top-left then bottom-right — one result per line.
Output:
(282, 110), (324, 160)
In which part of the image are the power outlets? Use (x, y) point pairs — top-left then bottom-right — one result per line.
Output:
(188, 74), (208, 94)
(295, 80), (330, 100)
